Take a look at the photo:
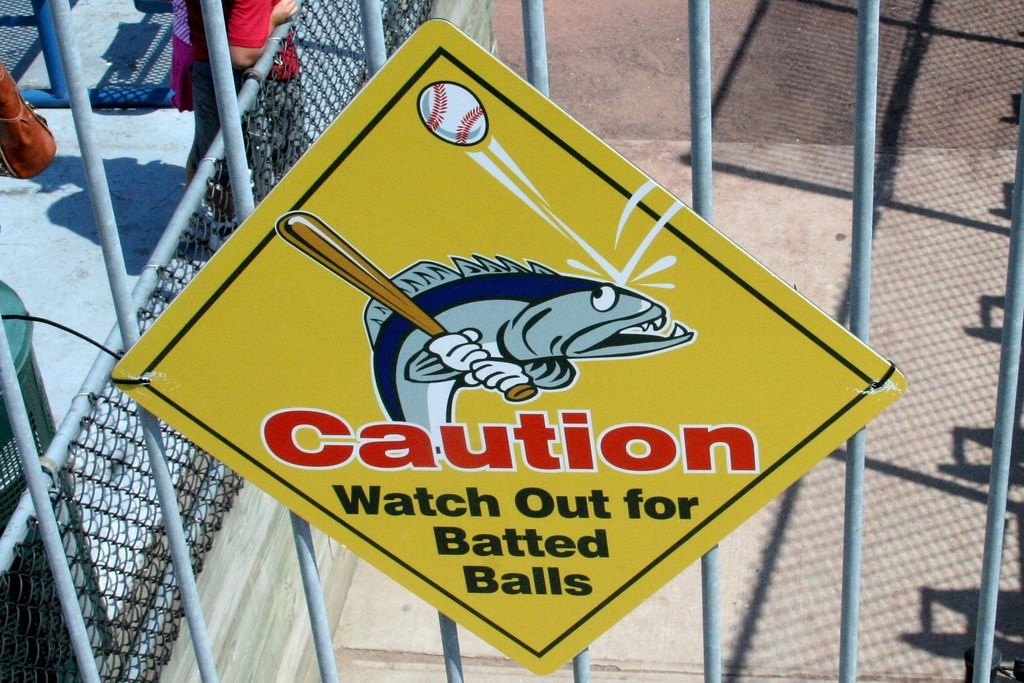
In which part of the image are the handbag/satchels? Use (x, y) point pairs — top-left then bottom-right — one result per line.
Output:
(0, 64), (56, 179)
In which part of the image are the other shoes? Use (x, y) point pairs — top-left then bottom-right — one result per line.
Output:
(179, 206), (213, 246)
(208, 217), (238, 253)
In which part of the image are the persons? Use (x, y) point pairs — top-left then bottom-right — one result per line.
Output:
(134, 0), (308, 251)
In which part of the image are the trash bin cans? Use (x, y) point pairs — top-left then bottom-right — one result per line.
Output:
(0, 279), (122, 683)
